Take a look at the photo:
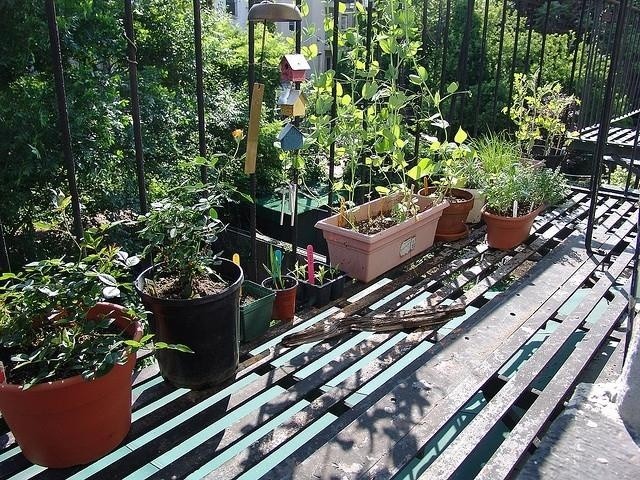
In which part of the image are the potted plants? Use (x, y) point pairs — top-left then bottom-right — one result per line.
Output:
(480, 165), (567, 252)
(313, 190), (450, 284)
(1, 256), (148, 469)
(287, 244), (350, 310)
(239, 280), (277, 345)
(261, 241), (299, 321)
(133, 196), (244, 392)
(415, 126), (475, 242)
(440, 130), (486, 225)
